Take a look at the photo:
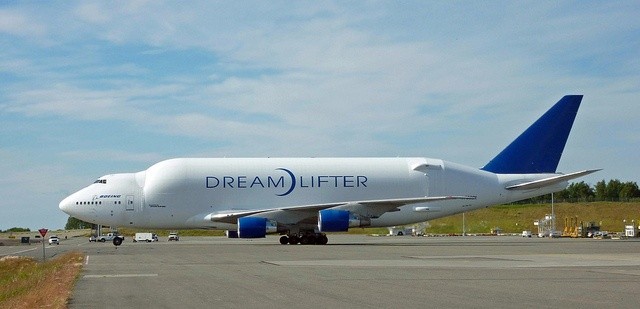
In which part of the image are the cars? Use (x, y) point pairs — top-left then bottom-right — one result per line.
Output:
(89, 236), (96, 242)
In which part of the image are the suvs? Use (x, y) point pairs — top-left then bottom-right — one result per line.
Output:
(49, 236), (59, 245)
(168, 233), (179, 241)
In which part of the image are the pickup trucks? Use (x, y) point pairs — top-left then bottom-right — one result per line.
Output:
(98, 233), (124, 242)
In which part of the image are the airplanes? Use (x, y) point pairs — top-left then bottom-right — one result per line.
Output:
(58, 95), (604, 245)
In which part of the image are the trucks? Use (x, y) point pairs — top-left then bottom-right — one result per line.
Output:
(135, 233), (158, 242)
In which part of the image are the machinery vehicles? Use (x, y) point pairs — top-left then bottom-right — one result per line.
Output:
(522, 215), (604, 241)
